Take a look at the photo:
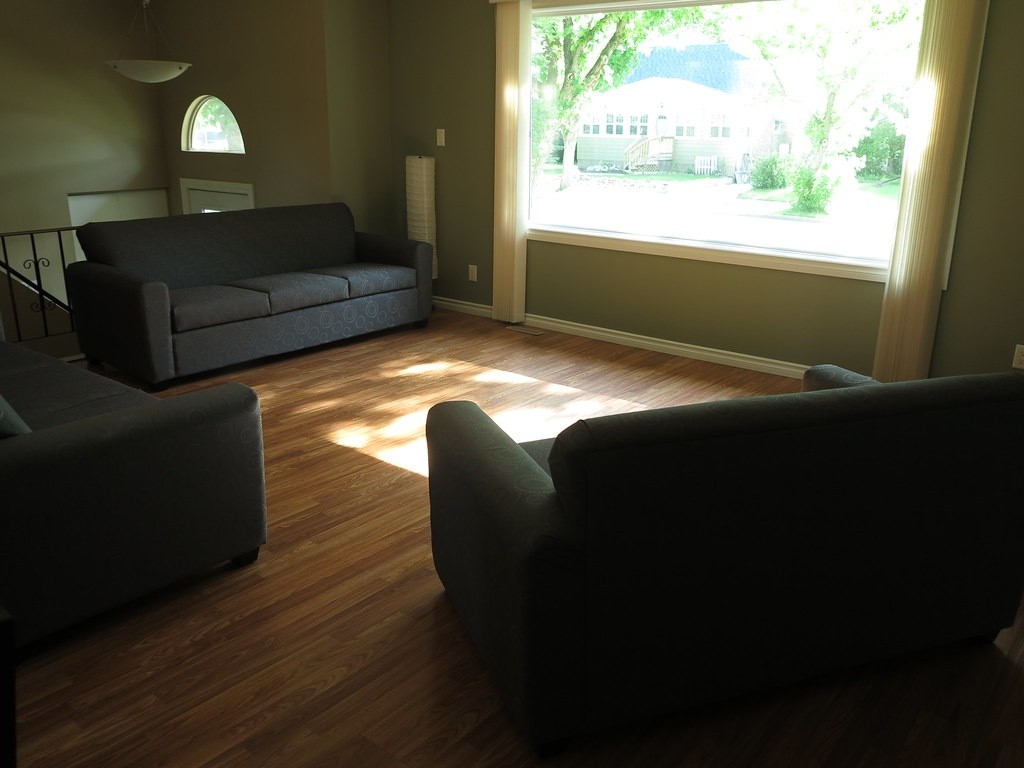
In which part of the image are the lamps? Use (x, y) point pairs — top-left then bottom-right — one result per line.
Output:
(105, 60), (193, 83)
(406, 155), (439, 279)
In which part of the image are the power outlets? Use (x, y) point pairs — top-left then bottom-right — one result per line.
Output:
(1012, 344), (1024, 371)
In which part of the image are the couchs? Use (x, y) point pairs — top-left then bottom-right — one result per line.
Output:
(427, 365), (1024, 749)
(0, 334), (269, 653)
(66, 202), (434, 391)
